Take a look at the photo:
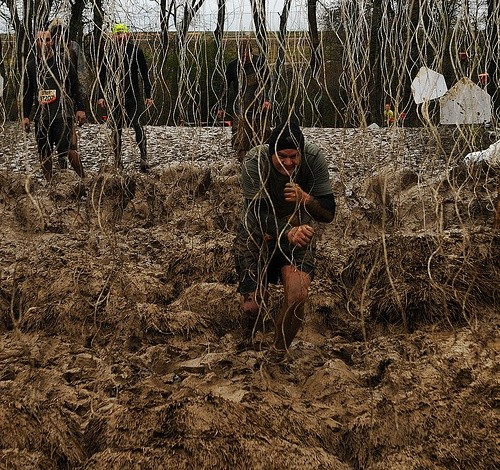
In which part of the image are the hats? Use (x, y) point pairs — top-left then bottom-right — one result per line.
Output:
(269, 123), (304, 158)
(113, 23), (129, 35)
(51, 18), (68, 33)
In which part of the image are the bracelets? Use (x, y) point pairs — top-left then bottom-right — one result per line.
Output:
(284, 225), (294, 237)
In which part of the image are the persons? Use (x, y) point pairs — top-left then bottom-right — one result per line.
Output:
(212, 108), (233, 127)
(22, 27), (88, 181)
(138, 97), (159, 127)
(94, 98), (118, 125)
(255, 102), (281, 126)
(384, 103), (393, 126)
(229, 121), (335, 352)
(47, 17), (88, 171)
(98, 24), (154, 172)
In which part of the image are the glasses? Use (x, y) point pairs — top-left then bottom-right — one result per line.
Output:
(36, 37), (51, 42)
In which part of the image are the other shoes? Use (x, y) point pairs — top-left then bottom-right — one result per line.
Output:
(140, 158), (149, 172)
(265, 360), (290, 378)
